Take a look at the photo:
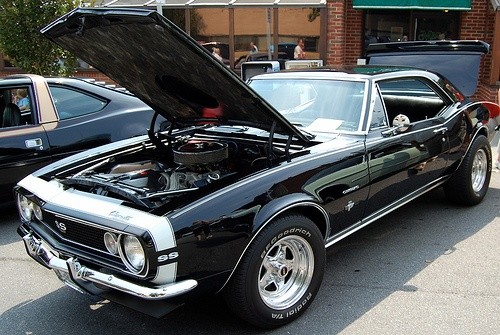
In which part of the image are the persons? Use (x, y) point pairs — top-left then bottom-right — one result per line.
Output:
(250, 42), (259, 61)
(294, 39), (306, 60)
(211, 47), (224, 64)
(192, 220), (213, 240)
(0, 102), (22, 127)
(11, 89), (30, 112)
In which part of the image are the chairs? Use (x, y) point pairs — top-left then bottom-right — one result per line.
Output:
(2, 103), (21, 128)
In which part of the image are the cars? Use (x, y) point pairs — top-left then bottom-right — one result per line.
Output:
(0, 70), (172, 208)
(14, 4), (493, 332)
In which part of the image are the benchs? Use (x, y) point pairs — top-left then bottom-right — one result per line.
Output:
(348, 94), (443, 128)
